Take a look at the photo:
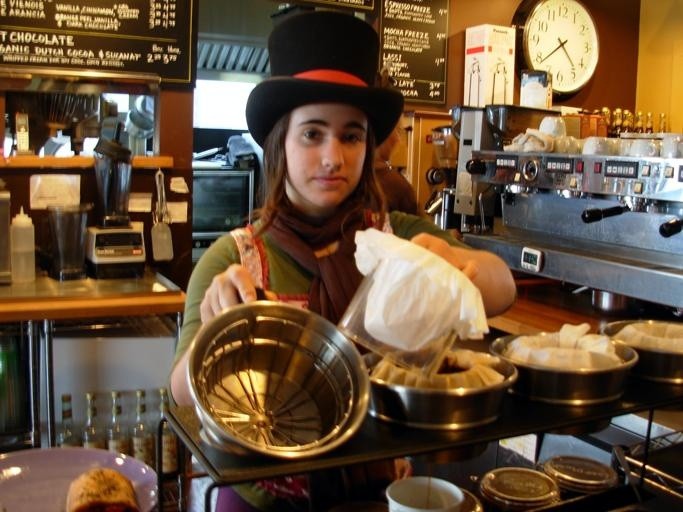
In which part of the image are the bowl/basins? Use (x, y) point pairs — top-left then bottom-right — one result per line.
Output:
(492, 330), (642, 408)
(357, 351), (520, 432)
(339, 260), (461, 376)
(590, 289), (637, 316)
(603, 320), (683, 386)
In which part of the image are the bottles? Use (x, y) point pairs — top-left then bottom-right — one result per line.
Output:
(55, 392), (78, 449)
(108, 389), (128, 456)
(128, 388), (152, 469)
(154, 386), (179, 480)
(581, 106), (668, 137)
(8, 206), (36, 285)
(79, 392), (102, 452)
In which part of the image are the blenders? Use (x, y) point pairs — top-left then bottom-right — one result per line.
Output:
(80, 133), (147, 281)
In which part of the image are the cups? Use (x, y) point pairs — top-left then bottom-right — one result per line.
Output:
(556, 133), (683, 157)
(539, 115), (566, 138)
(44, 203), (95, 282)
(519, 68), (553, 111)
(383, 476), (464, 512)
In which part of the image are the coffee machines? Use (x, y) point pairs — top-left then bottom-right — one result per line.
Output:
(460, 151), (682, 315)
(425, 125), (461, 228)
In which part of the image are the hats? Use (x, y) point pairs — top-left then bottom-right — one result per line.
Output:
(245, 11), (405, 149)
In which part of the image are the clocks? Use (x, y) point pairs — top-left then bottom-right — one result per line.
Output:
(511, 0), (599, 101)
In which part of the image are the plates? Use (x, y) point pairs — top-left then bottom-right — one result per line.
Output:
(1, 450), (162, 511)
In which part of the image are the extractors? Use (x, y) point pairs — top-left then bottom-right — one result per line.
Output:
(195, 37), (271, 83)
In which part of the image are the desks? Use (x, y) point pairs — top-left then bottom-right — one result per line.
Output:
(0, 268), (187, 448)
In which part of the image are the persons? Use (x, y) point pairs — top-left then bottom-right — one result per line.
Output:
(371, 110), (462, 240)
(166, 105), (519, 410)
